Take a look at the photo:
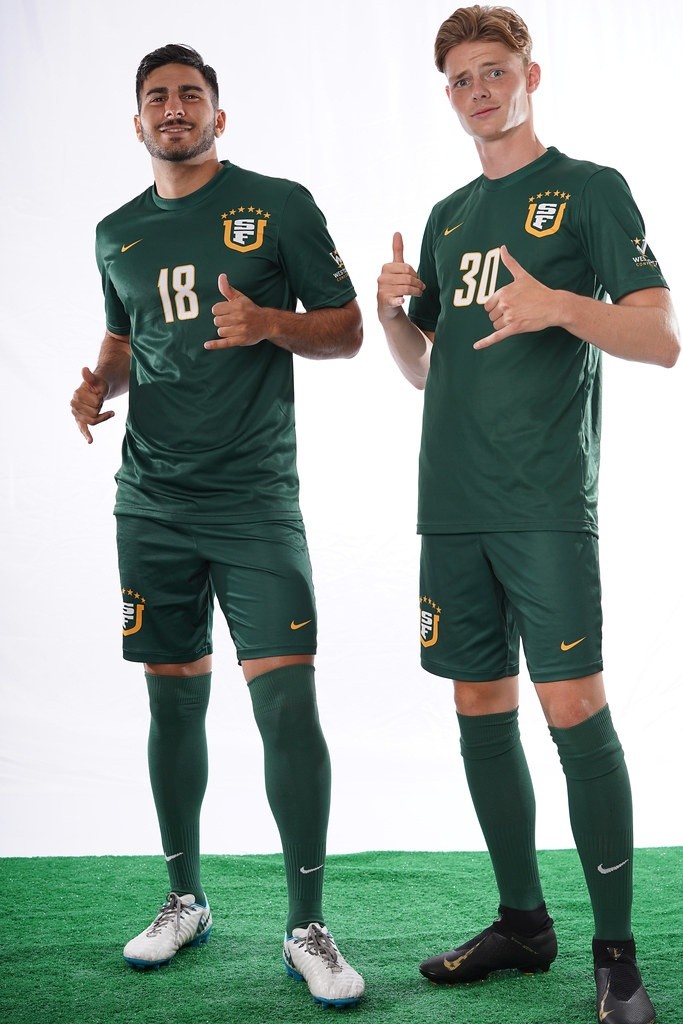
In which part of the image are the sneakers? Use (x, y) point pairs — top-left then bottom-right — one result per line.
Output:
(587, 935), (659, 1023)
(418, 924), (557, 984)
(123, 892), (212, 970)
(279, 923), (367, 1009)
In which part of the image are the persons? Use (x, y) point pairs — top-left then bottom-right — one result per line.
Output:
(375, 2), (682, 1024)
(69, 43), (372, 1007)
